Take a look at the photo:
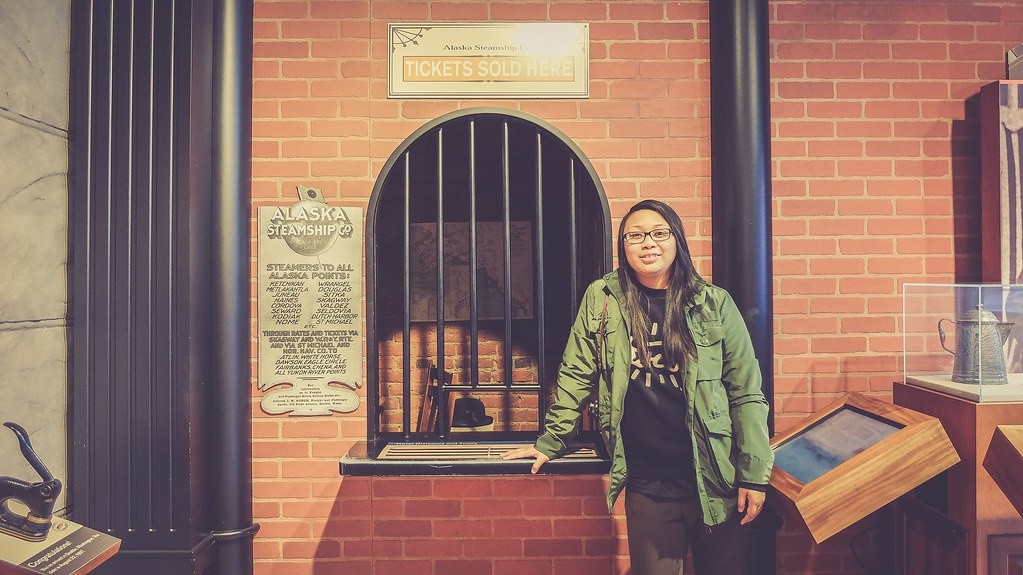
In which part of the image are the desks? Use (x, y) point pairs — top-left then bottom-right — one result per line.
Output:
(891, 380), (1022, 575)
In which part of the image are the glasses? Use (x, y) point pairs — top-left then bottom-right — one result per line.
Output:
(622, 228), (673, 244)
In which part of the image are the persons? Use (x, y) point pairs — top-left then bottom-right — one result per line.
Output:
(500, 200), (775, 574)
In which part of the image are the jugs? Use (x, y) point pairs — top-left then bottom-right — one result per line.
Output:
(939, 302), (1015, 386)
